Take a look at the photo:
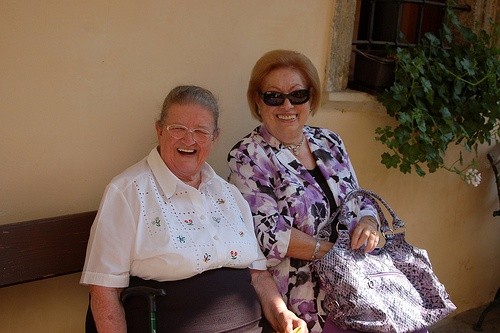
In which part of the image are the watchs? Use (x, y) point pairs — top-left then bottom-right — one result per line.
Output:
(359, 216), (378, 226)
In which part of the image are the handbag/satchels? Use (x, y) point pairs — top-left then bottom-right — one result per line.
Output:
(306, 188), (459, 333)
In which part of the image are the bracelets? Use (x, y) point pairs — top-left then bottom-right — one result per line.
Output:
(310, 237), (321, 262)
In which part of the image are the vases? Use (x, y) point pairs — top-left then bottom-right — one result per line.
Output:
(352, 47), (393, 94)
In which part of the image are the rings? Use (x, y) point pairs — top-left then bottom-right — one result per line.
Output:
(371, 230), (377, 236)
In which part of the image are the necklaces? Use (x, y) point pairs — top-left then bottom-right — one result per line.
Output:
(283, 133), (305, 156)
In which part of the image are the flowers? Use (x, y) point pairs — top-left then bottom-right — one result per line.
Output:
(375, 11), (500, 187)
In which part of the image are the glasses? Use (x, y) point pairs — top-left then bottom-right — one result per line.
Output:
(161, 119), (214, 143)
(257, 88), (313, 107)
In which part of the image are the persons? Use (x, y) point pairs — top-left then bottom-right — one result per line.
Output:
(227, 49), (381, 333)
(79, 85), (309, 333)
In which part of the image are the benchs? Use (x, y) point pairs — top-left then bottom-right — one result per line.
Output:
(0, 211), (97, 333)
